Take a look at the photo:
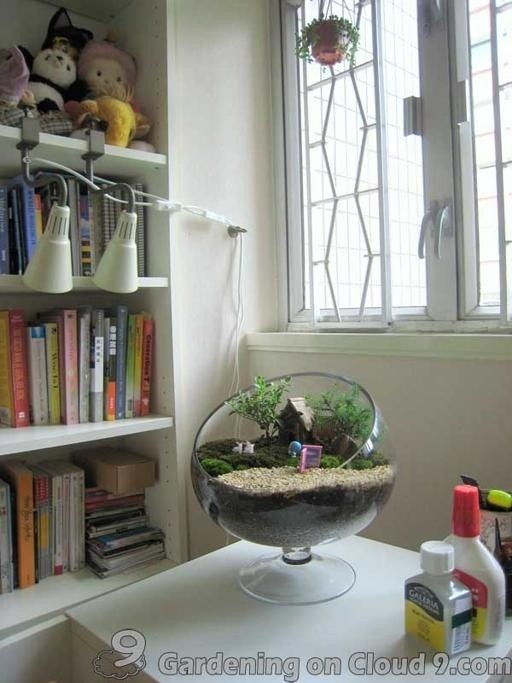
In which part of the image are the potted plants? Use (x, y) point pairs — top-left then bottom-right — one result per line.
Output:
(191, 371), (399, 604)
(294, 0), (359, 72)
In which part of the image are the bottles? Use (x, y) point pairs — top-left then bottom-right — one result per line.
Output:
(404, 478), (511, 657)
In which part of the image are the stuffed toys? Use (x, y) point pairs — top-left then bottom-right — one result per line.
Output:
(0, 7), (157, 152)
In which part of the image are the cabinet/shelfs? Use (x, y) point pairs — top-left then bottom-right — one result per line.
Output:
(2, 3), (179, 683)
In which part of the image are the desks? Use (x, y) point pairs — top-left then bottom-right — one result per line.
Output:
(63, 535), (512, 682)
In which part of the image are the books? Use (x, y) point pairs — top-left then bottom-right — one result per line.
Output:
(1, 459), (165, 593)
(0, 306), (154, 428)
(1, 173), (145, 276)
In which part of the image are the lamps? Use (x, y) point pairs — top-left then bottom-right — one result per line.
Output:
(81, 128), (139, 293)
(12, 115), (74, 293)
